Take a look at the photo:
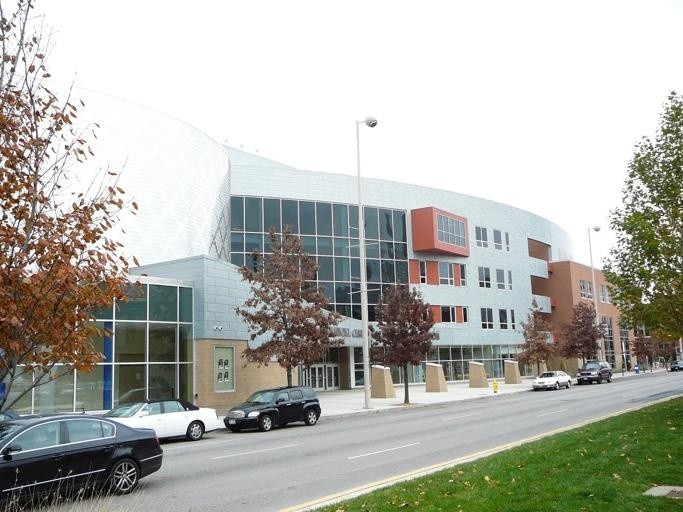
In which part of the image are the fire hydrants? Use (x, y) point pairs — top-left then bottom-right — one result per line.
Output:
(492, 378), (499, 393)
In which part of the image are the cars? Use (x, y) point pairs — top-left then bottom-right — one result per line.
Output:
(670, 359), (683, 372)
(10, 379), (96, 402)
(115, 386), (174, 404)
(0, 397), (218, 511)
(531, 371), (572, 390)
(223, 385), (321, 432)
(575, 360), (613, 384)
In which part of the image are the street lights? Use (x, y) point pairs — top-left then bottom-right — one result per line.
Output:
(355, 117), (377, 410)
(587, 225), (602, 360)
